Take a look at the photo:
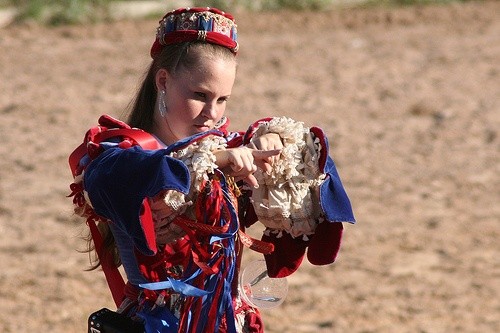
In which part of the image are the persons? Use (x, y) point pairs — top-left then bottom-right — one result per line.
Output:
(69, 7), (356, 333)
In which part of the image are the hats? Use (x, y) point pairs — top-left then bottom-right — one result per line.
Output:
(150, 8), (239, 58)
